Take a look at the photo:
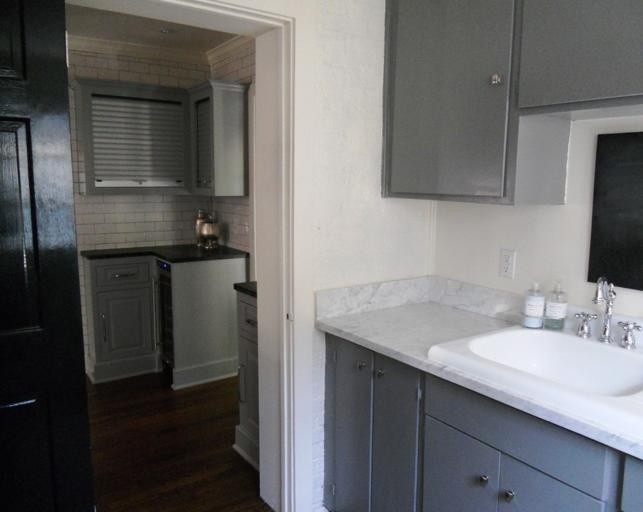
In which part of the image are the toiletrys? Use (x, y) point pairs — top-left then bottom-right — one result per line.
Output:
(545, 283), (569, 330)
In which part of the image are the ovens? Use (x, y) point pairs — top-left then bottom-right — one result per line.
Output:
(149, 260), (173, 368)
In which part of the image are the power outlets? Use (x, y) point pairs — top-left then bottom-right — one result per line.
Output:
(499, 249), (517, 278)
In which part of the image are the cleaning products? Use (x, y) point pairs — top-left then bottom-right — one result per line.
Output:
(521, 281), (544, 327)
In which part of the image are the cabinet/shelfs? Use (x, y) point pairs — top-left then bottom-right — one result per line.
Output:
(421, 372), (615, 512)
(233, 290), (259, 476)
(75, 77), (249, 198)
(88, 256), (159, 381)
(517, 0), (643, 116)
(325, 333), (421, 512)
(382, 0), (518, 205)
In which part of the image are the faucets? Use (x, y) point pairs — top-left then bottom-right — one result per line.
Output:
(592, 275), (617, 344)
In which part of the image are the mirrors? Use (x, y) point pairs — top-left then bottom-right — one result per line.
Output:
(588, 132), (643, 290)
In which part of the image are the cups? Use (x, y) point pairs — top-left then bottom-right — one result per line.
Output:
(194, 207), (218, 249)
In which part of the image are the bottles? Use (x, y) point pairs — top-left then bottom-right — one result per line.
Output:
(521, 283), (568, 330)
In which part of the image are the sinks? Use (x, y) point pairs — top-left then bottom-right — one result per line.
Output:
(425, 326), (643, 406)
